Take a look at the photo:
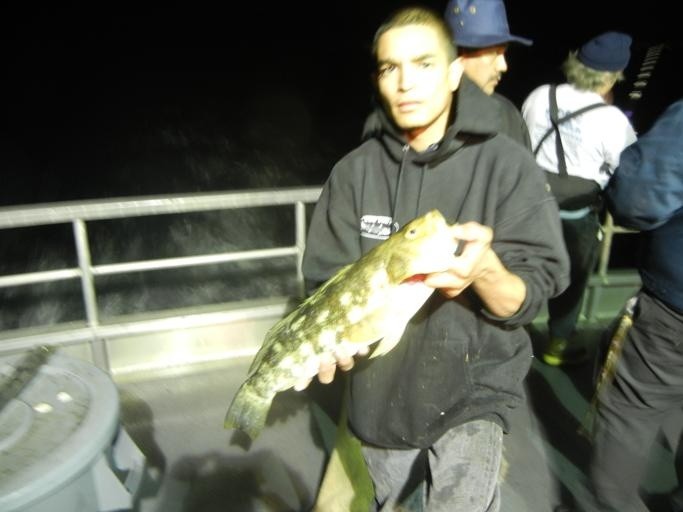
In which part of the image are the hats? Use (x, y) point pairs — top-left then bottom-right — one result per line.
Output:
(576, 31), (634, 72)
(443, 0), (533, 51)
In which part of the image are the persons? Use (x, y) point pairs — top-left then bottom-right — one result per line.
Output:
(361, 0), (535, 153)
(519, 29), (641, 368)
(301, 7), (573, 510)
(588, 100), (681, 512)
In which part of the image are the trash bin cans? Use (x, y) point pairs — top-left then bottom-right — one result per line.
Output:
(0, 347), (146, 512)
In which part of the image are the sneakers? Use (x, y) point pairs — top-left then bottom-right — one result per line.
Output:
(540, 330), (597, 366)
